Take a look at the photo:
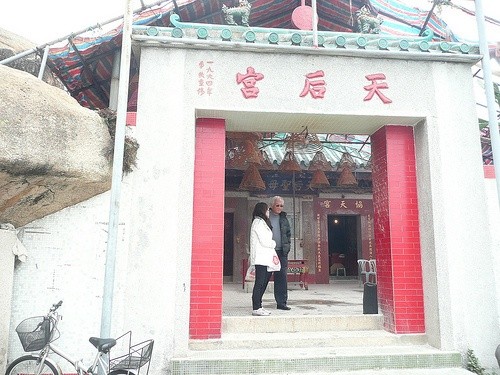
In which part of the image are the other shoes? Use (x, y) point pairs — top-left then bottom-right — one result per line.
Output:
(277, 306), (291, 310)
(252, 308), (270, 316)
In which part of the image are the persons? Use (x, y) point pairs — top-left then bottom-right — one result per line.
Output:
(268, 196), (291, 310)
(249, 202), (276, 316)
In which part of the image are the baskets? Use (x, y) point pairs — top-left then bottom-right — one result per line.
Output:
(15, 316), (50, 352)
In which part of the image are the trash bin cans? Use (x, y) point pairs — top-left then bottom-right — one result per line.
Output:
(362, 282), (378, 314)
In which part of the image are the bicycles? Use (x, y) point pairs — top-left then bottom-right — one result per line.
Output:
(5, 300), (154, 375)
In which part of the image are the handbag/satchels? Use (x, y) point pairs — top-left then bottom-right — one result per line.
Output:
(245, 265), (256, 281)
(266, 247), (281, 271)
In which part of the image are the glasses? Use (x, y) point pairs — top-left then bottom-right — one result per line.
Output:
(274, 204), (283, 207)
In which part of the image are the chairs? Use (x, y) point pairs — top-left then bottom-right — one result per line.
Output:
(357, 258), (377, 288)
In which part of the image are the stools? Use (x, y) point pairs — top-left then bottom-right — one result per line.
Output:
(336, 267), (346, 277)
